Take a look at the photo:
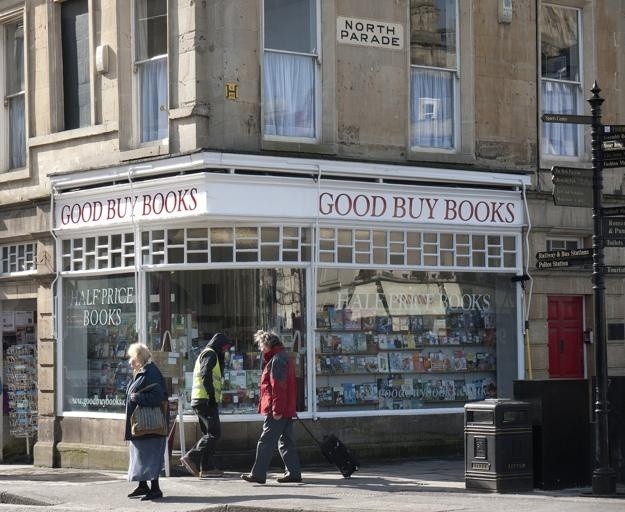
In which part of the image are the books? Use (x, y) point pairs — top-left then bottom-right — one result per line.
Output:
(316, 309), (497, 411)
(1, 311), (38, 438)
(86, 309), (309, 411)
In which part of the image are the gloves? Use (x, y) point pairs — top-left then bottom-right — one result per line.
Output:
(207, 398), (218, 410)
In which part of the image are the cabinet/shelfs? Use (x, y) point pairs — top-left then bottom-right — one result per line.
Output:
(87, 307), (498, 411)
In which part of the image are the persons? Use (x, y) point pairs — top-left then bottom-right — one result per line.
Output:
(240, 330), (302, 484)
(180, 332), (232, 478)
(123, 343), (169, 500)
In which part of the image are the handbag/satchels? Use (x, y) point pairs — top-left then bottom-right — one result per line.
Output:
(289, 351), (304, 379)
(151, 350), (186, 379)
(129, 404), (168, 438)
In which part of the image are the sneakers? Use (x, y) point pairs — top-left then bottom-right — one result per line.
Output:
(180, 456), (202, 476)
(141, 488), (163, 501)
(200, 467), (224, 478)
(128, 485), (151, 498)
(241, 474), (266, 484)
(277, 474), (302, 483)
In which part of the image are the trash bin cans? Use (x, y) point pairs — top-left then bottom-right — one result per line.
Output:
(464, 399), (533, 493)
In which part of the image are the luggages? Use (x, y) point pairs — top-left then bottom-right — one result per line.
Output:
(296, 416), (360, 480)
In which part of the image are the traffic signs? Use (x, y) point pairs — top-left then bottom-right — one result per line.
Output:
(603, 206), (625, 247)
(602, 124), (625, 142)
(604, 265), (625, 275)
(553, 166), (594, 208)
(604, 150), (625, 168)
(541, 113), (594, 124)
(535, 248), (592, 270)
